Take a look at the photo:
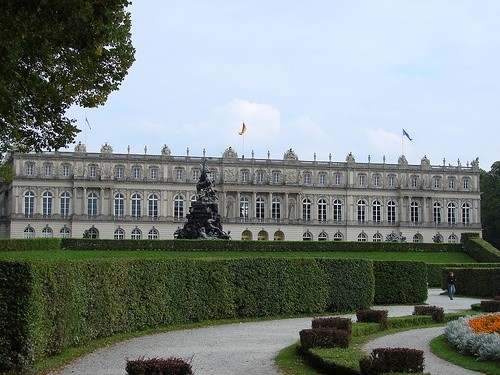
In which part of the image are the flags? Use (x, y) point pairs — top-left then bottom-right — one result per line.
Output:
(403, 129), (413, 141)
(238, 124), (247, 135)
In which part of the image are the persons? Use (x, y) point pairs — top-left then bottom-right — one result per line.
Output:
(447, 272), (456, 300)
